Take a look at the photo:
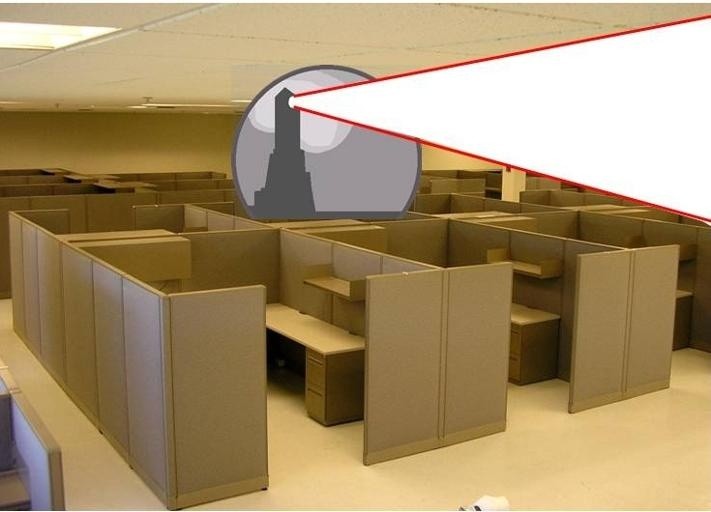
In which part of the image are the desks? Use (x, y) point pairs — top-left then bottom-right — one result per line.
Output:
(507, 301), (564, 385)
(242, 301), (365, 426)
